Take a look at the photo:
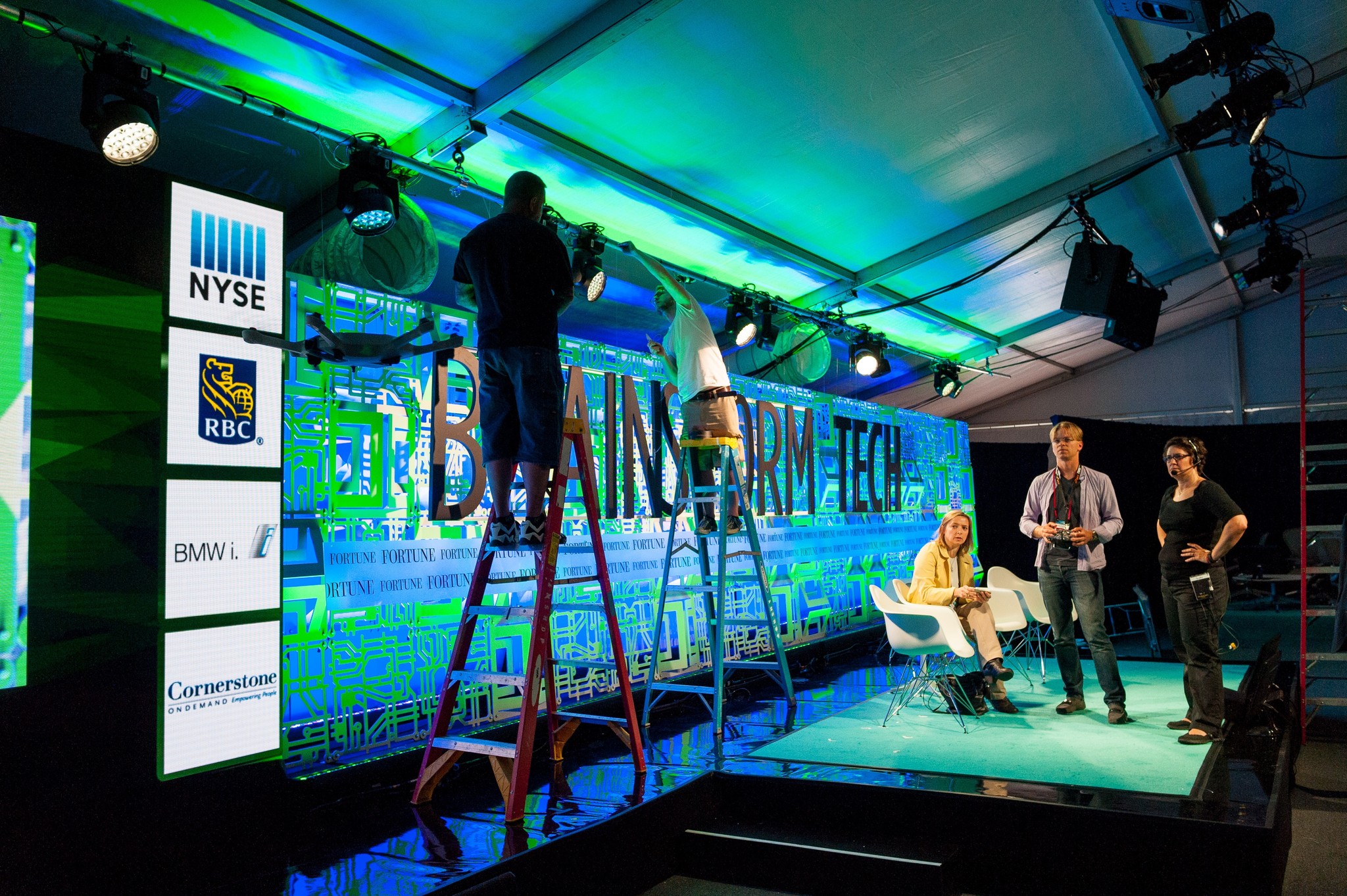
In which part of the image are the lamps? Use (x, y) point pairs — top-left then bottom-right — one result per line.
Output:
(341, 143), (395, 235)
(849, 334), (891, 379)
(1062, 5), (1313, 356)
(84, 43), (163, 166)
(565, 235), (609, 299)
(930, 360), (965, 399)
(724, 297), (778, 351)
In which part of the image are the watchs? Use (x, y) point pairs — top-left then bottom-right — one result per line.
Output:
(1091, 529), (1099, 541)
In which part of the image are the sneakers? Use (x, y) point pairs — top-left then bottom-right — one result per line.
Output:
(518, 520), (567, 544)
(727, 515), (743, 534)
(1107, 704), (1128, 724)
(1056, 696), (1086, 714)
(489, 520), (522, 547)
(693, 516), (717, 535)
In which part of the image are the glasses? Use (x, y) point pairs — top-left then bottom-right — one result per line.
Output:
(1163, 454), (1192, 463)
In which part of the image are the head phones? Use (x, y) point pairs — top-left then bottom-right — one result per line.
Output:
(1187, 438), (1202, 471)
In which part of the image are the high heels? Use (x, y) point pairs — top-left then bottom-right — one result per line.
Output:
(987, 694), (1019, 714)
(986, 658), (1013, 684)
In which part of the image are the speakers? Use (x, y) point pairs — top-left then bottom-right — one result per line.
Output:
(1057, 242), (1133, 318)
(1101, 277), (1164, 355)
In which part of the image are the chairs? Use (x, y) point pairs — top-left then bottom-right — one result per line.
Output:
(868, 564), (1076, 721)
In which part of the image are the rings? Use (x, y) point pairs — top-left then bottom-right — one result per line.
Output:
(963, 592), (968, 597)
(1191, 552), (1195, 556)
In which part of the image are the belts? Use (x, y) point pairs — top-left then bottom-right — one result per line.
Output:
(689, 386), (737, 401)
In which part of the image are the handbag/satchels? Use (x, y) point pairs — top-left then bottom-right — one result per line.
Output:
(924, 671), (990, 716)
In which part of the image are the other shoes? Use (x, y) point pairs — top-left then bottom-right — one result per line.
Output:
(1177, 728), (1223, 744)
(1167, 720), (1191, 730)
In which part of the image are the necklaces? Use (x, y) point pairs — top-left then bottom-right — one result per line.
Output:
(949, 555), (956, 573)
(1177, 476), (1201, 498)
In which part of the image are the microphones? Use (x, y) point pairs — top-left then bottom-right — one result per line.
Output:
(1170, 460), (1202, 476)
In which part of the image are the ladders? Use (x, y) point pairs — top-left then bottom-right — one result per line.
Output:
(638, 435), (798, 737)
(410, 366), (648, 826)
(1297, 281), (1347, 746)
(1099, 602), (1148, 639)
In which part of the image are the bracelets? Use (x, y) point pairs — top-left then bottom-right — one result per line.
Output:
(1206, 550), (1216, 564)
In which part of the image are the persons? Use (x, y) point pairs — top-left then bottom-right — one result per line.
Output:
(1020, 419), (1130, 725)
(618, 239), (746, 535)
(452, 171), (574, 546)
(1153, 436), (1249, 745)
(906, 508), (1020, 714)
